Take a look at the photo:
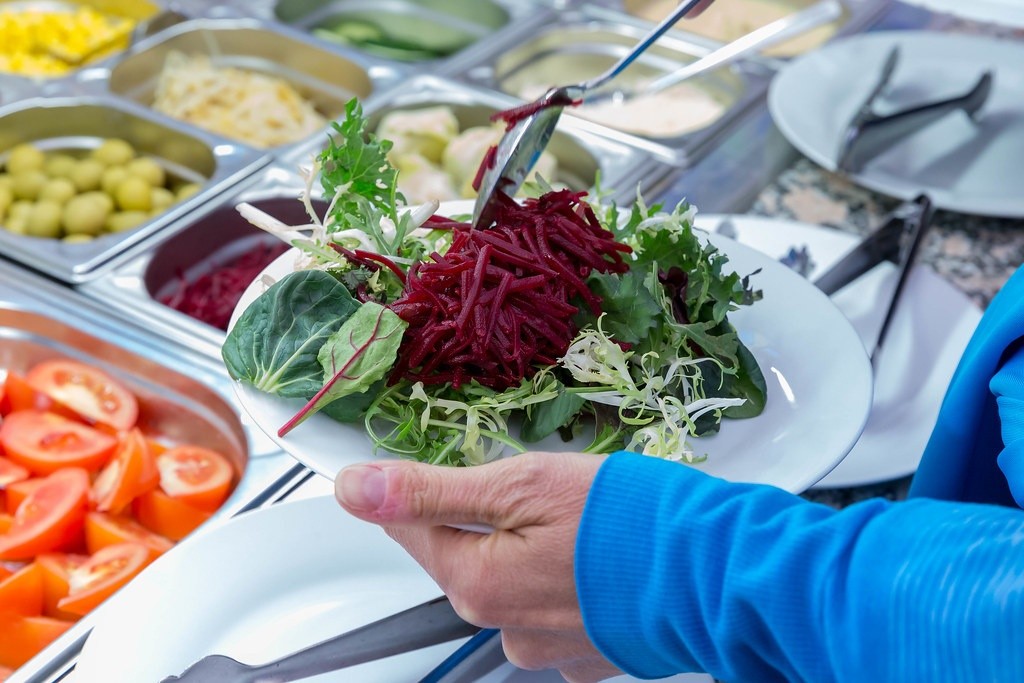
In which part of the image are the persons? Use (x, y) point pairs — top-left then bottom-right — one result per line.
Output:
(336, 0), (1024, 683)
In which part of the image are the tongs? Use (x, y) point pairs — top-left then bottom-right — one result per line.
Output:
(833, 41), (992, 176)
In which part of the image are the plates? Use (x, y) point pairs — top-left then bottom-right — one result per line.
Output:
(674, 213), (988, 491)
(72, 493), (715, 683)
(224, 198), (877, 530)
(769, 27), (1024, 224)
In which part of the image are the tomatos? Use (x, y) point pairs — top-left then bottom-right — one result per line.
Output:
(0, 358), (234, 683)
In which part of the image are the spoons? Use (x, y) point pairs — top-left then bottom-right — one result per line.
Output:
(468, 0), (702, 227)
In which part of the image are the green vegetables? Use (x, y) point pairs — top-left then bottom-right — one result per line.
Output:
(220, 98), (769, 476)
